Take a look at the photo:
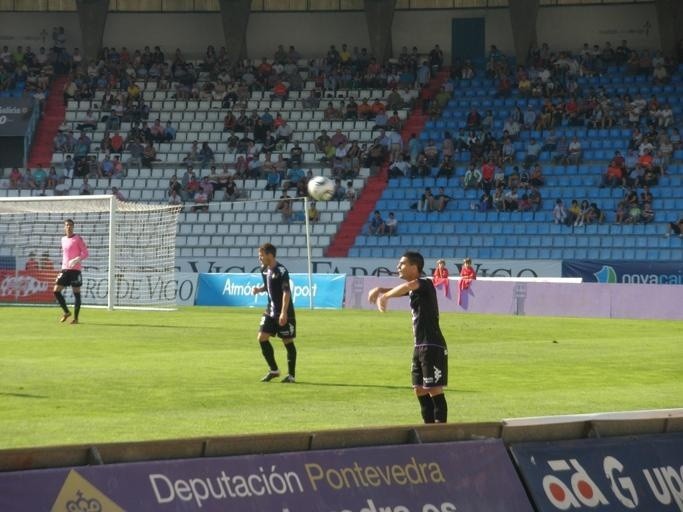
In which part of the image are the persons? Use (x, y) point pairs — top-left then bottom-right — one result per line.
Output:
(0, 28), (683, 240)
(40, 252), (53, 269)
(252, 243), (296, 384)
(24, 253), (37, 269)
(369, 251), (448, 423)
(460, 257), (475, 279)
(431, 259), (446, 278)
(51, 219), (88, 324)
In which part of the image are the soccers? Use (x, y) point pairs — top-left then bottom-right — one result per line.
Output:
(307, 175), (335, 202)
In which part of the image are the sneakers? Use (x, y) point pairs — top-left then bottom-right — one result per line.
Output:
(60, 311), (70, 322)
(70, 320), (77, 324)
(280, 374), (294, 382)
(260, 372), (279, 382)
